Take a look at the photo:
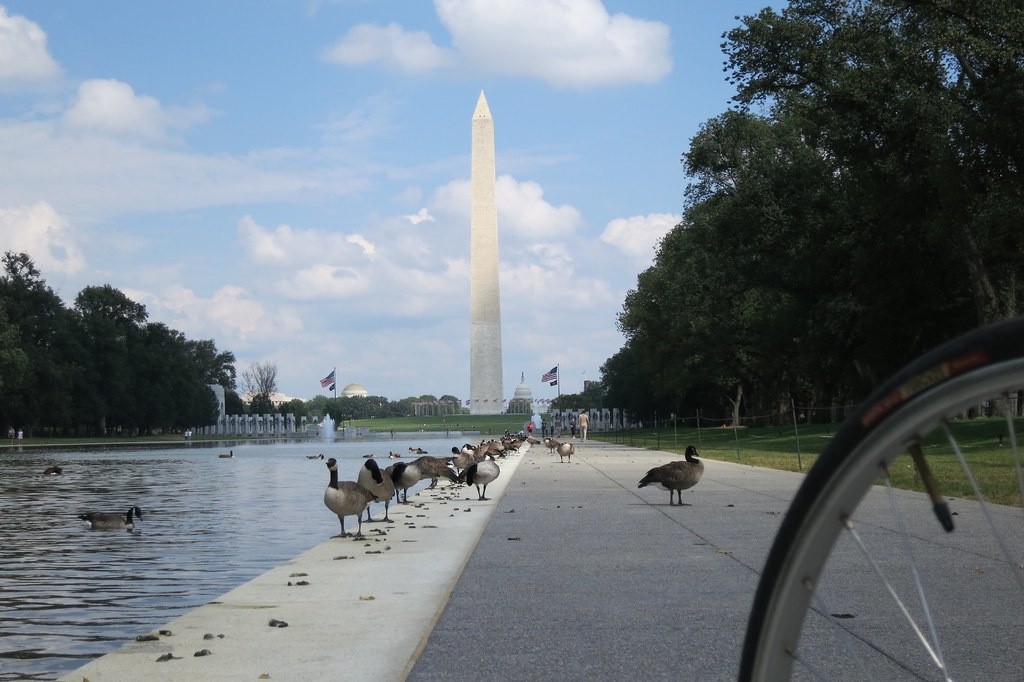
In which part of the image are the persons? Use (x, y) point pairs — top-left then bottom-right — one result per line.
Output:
(550, 424), (555, 437)
(7, 426), (15, 445)
(540, 420), (548, 438)
(527, 421), (534, 436)
(569, 418), (577, 439)
(184, 429), (192, 443)
(578, 410), (590, 443)
(16, 429), (24, 444)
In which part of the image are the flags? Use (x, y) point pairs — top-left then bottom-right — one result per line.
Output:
(329, 384), (335, 391)
(549, 380), (558, 386)
(541, 366), (558, 382)
(320, 371), (335, 388)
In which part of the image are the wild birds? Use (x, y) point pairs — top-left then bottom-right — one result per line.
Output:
(466, 451), (500, 500)
(219, 450), (234, 458)
(557, 442), (575, 463)
(543, 437), (560, 453)
(384, 462), (422, 504)
(42, 466), (63, 476)
(356, 459), (395, 523)
(388, 452), (400, 459)
(324, 458), (380, 538)
(404, 430), (541, 490)
(77, 506), (144, 529)
(306, 454), (324, 460)
(637, 445), (704, 506)
(362, 454), (374, 458)
